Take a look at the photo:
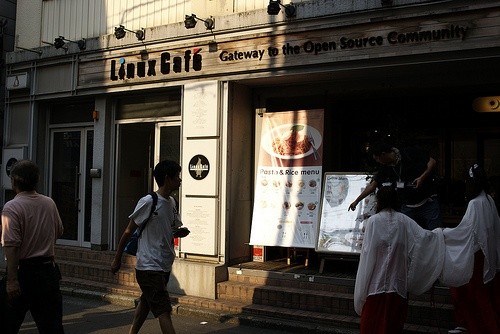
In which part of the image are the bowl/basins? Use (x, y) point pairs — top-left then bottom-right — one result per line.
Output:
(262, 123), (322, 160)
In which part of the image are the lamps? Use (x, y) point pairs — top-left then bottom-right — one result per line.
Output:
(113, 25), (147, 43)
(54, 33), (87, 52)
(266, 0), (297, 18)
(185, 13), (220, 33)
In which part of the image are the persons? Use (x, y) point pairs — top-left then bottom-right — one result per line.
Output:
(110, 160), (190, 334)
(0, 159), (64, 334)
(348, 143), (500, 334)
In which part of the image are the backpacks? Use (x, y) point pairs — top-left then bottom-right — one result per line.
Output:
(123, 191), (179, 256)
